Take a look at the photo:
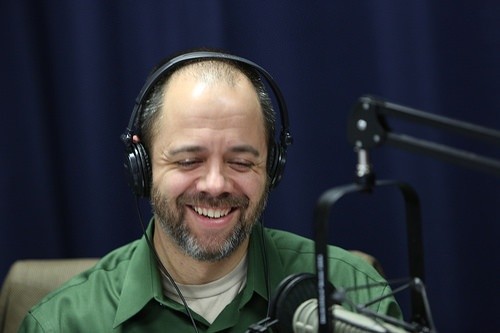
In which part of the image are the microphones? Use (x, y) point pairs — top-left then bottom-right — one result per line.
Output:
(271, 273), (411, 333)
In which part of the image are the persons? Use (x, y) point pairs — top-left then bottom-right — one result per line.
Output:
(16, 49), (404, 333)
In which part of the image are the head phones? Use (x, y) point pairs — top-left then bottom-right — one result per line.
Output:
(117, 53), (293, 201)
